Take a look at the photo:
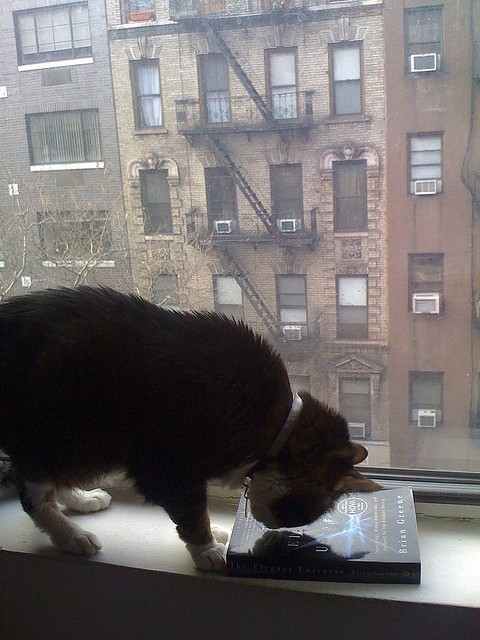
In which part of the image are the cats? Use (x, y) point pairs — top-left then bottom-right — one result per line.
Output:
(0, 283), (383, 573)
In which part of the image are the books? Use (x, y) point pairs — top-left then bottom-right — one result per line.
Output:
(222, 479), (422, 583)
